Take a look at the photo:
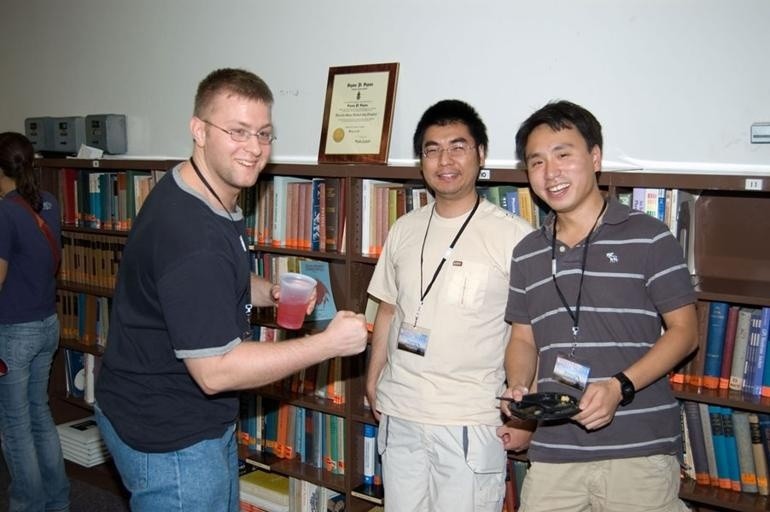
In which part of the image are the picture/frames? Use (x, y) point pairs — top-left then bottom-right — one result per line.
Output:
(315, 61), (401, 165)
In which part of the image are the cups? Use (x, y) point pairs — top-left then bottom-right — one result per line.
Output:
(275, 271), (318, 331)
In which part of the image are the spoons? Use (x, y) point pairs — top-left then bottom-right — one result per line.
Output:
(494, 395), (573, 416)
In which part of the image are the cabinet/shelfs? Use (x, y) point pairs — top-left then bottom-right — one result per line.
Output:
(349, 164), (611, 510)
(612, 173), (769, 511)
(166, 160), (349, 512)
(33, 157), (167, 512)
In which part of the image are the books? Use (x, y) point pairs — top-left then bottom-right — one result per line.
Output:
(235, 172), (347, 512)
(360, 180), (434, 512)
(474, 183), (547, 229)
(668, 300), (769, 497)
(37, 166), (165, 470)
(619, 188), (700, 291)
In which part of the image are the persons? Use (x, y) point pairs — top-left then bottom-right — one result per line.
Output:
(95, 67), (367, 511)
(0, 130), (72, 511)
(364, 99), (537, 512)
(502, 97), (700, 512)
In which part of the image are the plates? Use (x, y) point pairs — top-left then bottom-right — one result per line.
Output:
(508, 390), (581, 422)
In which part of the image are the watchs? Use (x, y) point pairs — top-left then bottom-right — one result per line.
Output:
(611, 372), (635, 407)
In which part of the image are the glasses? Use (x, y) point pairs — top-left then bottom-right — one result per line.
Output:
(202, 119), (277, 145)
(422, 144), (476, 159)
